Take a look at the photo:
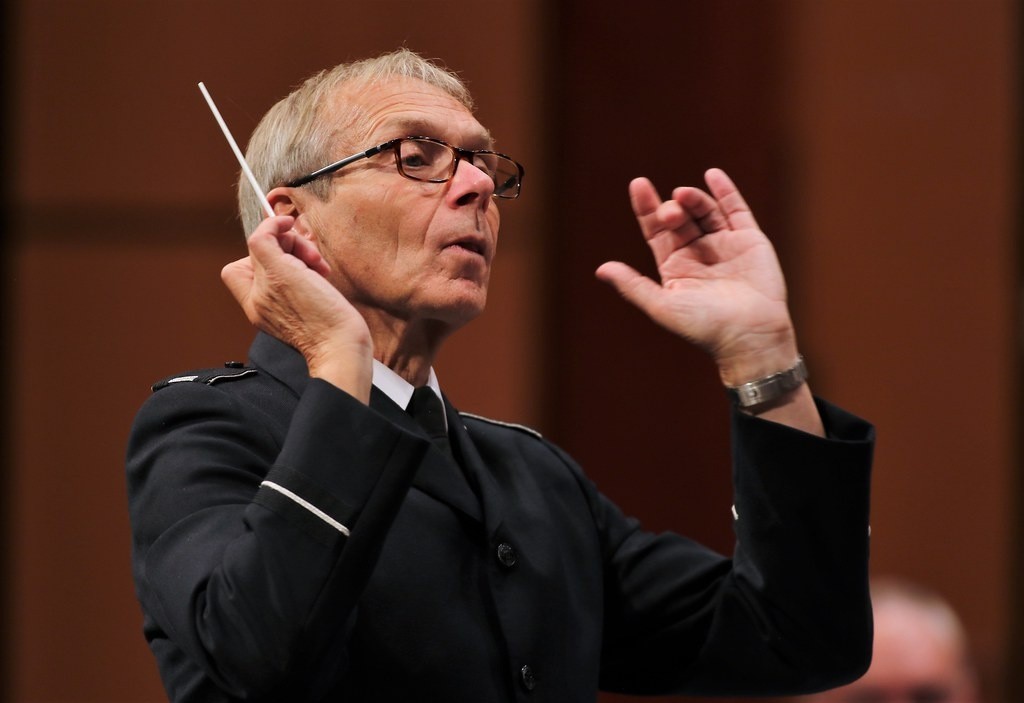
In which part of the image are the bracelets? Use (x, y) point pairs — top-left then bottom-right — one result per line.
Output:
(724, 355), (809, 408)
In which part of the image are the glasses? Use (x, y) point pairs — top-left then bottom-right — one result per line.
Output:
(287, 136), (525, 200)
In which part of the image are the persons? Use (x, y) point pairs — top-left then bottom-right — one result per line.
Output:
(126, 50), (874, 703)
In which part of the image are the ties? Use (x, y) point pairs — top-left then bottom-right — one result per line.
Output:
(406, 386), (473, 490)
